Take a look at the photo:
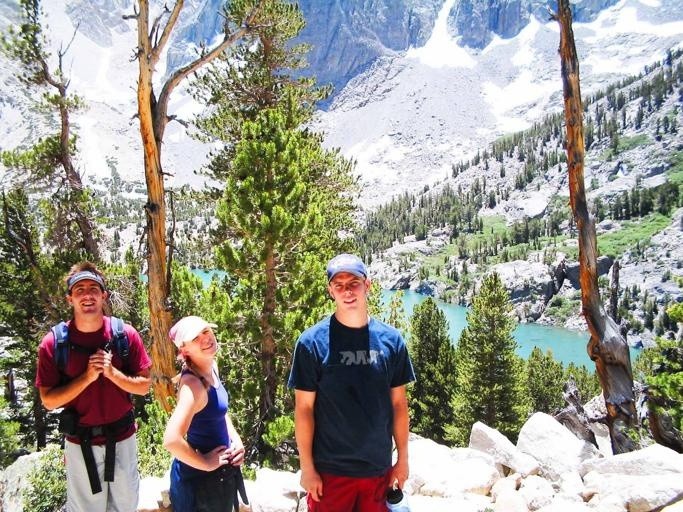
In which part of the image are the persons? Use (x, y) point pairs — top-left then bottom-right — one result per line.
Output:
(164, 316), (244, 512)
(288, 254), (415, 512)
(36, 261), (152, 512)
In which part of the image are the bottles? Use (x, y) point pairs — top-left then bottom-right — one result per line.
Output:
(386, 489), (410, 512)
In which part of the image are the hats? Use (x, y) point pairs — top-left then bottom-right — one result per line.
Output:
(327, 253), (367, 284)
(168, 316), (218, 349)
(68, 271), (107, 292)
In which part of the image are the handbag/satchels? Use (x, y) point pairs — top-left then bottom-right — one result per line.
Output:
(193, 460), (249, 512)
